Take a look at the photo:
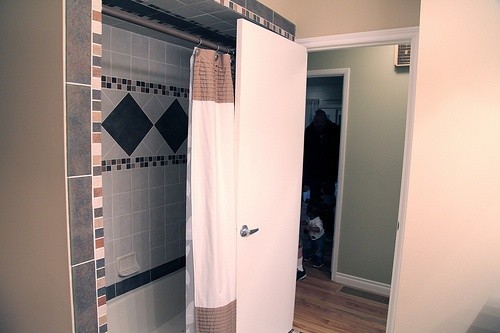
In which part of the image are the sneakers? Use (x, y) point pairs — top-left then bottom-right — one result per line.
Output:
(296, 266), (306, 281)
(304, 256), (311, 261)
(312, 263), (324, 268)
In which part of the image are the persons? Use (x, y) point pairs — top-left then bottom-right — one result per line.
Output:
(304, 109), (342, 251)
(304, 189), (334, 268)
